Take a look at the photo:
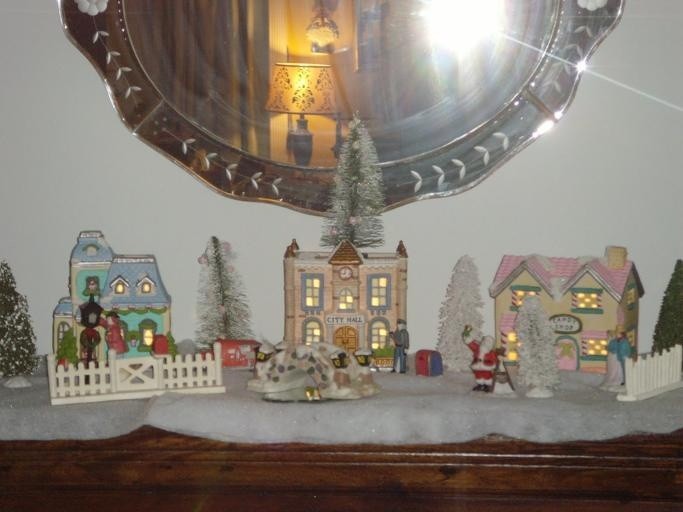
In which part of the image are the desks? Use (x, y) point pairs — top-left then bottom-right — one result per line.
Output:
(0, 358), (683, 512)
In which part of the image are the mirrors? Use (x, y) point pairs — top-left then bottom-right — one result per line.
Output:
(58, 0), (624, 218)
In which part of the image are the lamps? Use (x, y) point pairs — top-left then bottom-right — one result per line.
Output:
(262, 62), (343, 167)
